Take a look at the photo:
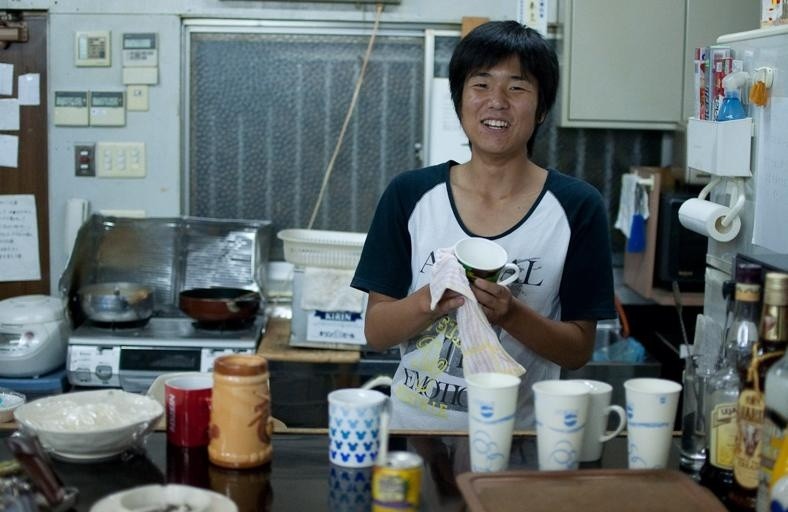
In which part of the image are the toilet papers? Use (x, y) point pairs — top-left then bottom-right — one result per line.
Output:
(677, 196), (741, 242)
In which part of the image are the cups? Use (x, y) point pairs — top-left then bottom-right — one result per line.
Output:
(453, 237), (521, 292)
(464, 371), (682, 471)
(163, 374), (223, 449)
(326, 386), (390, 469)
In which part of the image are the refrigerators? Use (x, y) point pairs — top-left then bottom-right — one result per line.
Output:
(692, 26), (786, 511)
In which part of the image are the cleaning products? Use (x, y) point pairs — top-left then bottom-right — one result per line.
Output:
(716, 71), (751, 121)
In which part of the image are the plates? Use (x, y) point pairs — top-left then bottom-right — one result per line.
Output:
(87, 484), (240, 512)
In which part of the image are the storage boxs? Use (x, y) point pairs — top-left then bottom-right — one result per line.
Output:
(290, 263), (367, 350)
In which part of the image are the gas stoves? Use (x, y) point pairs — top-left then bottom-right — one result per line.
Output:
(64, 315), (272, 392)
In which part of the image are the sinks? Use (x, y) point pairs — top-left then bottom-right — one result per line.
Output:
(560, 322), (674, 388)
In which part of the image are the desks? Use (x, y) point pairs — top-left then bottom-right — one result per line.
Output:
(257, 318), (358, 430)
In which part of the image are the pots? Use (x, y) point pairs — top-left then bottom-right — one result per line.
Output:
(77, 280), (155, 324)
(178, 285), (261, 325)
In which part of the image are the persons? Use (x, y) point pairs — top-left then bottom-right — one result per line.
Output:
(350, 20), (617, 430)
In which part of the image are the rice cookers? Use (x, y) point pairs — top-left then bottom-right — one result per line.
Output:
(0, 293), (74, 380)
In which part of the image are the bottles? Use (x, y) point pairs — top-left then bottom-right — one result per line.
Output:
(0, 461), (39, 512)
(208, 353), (276, 470)
(680, 261), (787, 512)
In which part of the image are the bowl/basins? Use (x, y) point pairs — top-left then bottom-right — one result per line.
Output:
(13, 388), (165, 461)
(0, 387), (27, 423)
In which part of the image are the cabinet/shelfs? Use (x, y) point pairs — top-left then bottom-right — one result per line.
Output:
(555, 0), (761, 130)
